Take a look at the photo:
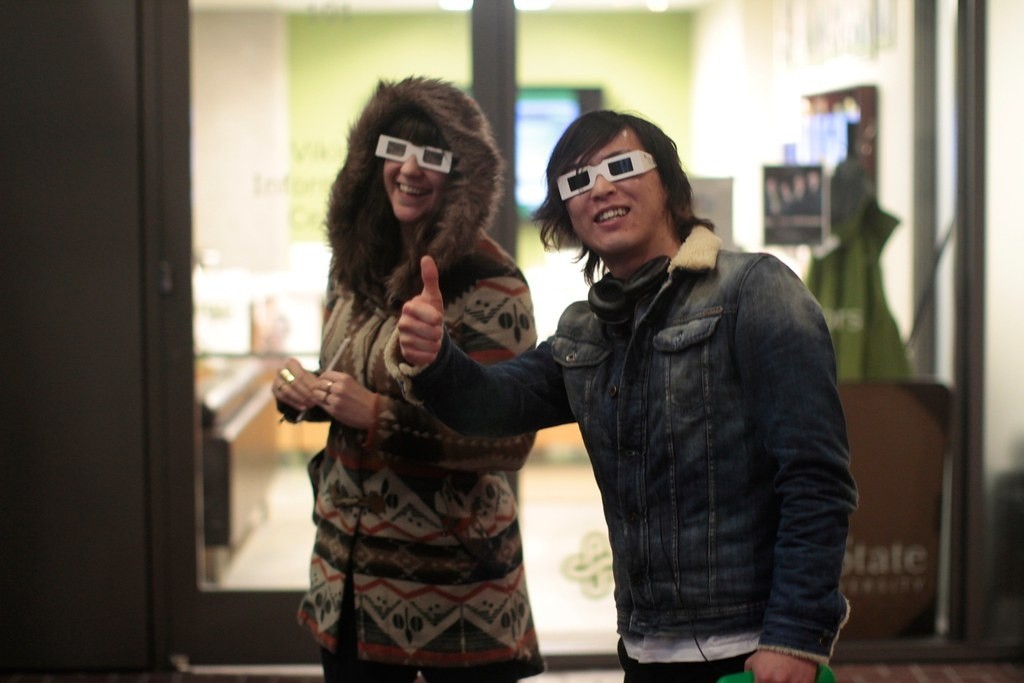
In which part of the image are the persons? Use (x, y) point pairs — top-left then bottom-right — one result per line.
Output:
(398, 110), (859, 683)
(271, 77), (538, 682)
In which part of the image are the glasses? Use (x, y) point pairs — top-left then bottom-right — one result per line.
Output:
(374, 135), (453, 176)
(557, 150), (655, 200)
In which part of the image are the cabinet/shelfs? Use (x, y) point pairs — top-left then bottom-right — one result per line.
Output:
(197, 359), (275, 582)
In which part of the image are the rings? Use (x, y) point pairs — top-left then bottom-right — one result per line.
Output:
(277, 382), (286, 390)
(322, 393), (331, 406)
(280, 368), (296, 383)
(326, 381), (333, 393)
